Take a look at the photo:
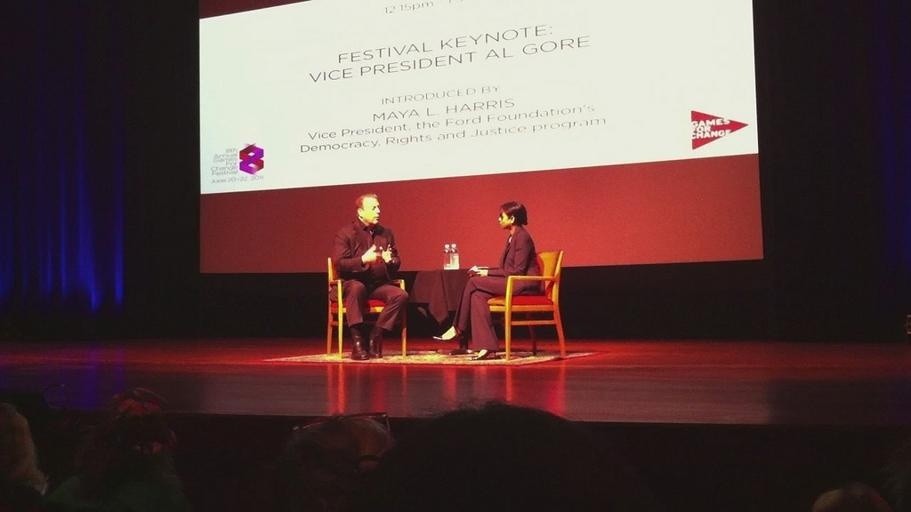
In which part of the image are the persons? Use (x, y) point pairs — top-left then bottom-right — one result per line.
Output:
(1, 381), (910, 511)
(333, 192), (413, 363)
(429, 200), (543, 359)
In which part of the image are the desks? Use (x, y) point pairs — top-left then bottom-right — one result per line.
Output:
(392, 267), (501, 355)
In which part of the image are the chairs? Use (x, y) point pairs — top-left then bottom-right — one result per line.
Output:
(487, 249), (566, 360)
(325, 257), (409, 358)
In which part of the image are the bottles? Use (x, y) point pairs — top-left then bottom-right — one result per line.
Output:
(443, 243), (460, 271)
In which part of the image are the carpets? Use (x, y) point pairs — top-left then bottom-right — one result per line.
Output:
(263, 350), (607, 366)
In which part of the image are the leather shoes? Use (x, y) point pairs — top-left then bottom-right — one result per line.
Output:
(368, 326), (383, 358)
(351, 346), (369, 360)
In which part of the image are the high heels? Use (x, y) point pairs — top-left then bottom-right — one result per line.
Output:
(432, 332), (464, 342)
(472, 349), (497, 360)
